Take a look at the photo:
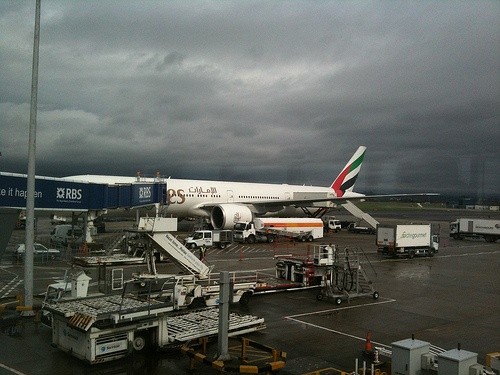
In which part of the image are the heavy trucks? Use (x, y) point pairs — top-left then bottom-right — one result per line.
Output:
(232, 217), (324, 245)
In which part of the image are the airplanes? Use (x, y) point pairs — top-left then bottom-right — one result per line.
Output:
(60, 145), (441, 234)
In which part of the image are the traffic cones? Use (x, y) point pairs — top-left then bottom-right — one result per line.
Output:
(362, 332), (373, 355)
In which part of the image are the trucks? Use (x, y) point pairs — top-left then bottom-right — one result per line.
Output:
(449, 218), (500, 243)
(324, 219), (342, 233)
(375, 223), (441, 259)
(346, 223), (374, 235)
(182, 228), (234, 250)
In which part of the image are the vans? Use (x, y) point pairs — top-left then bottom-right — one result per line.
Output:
(48, 224), (83, 246)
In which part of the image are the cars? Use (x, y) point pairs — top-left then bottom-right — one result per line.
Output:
(15, 242), (61, 262)
